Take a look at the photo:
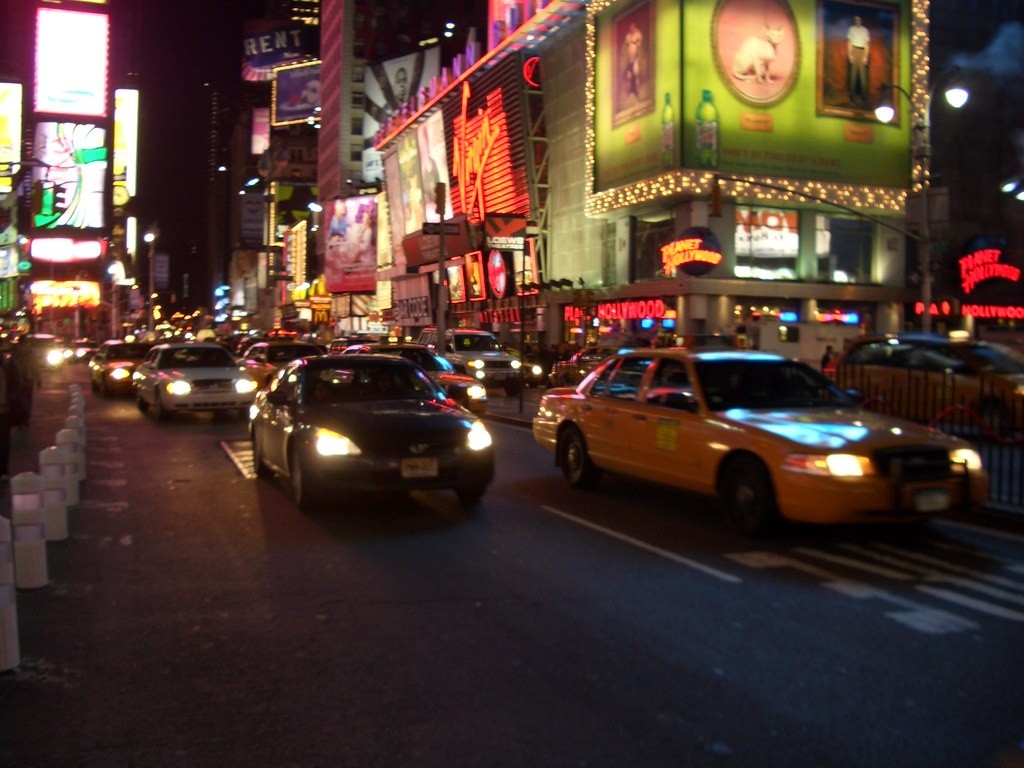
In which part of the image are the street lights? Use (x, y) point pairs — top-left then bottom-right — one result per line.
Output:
(144, 228), (155, 340)
(873, 67), (970, 327)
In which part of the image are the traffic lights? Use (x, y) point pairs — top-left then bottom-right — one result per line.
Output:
(30, 181), (43, 217)
(52, 182), (68, 214)
(708, 184), (721, 218)
(17, 241), (32, 274)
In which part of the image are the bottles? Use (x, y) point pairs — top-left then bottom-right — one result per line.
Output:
(693, 89), (721, 167)
(660, 92), (674, 166)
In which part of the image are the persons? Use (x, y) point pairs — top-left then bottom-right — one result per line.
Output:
(383, 69), (410, 113)
(537, 344), (558, 371)
(327, 199), (376, 274)
(4, 336), (42, 427)
(846, 14), (871, 106)
(821, 345), (840, 368)
(620, 22), (643, 104)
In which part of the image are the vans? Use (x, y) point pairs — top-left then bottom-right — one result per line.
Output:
(66, 337), (99, 362)
(26, 332), (66, 375)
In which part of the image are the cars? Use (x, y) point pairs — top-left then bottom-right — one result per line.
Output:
(234, 336), (329, 358)
(235, 341), (326, 390)
(339, 342), (488, 418)
(530, 347), (988, 546)
(548, 334), (649, 387)
(249, 353), (499, 515)
(130, 342), (256, 423)
(86, 339), (154, 399)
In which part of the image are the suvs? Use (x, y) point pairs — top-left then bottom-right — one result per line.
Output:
(819, 331), (1024, 443)
(415, 325), (524, 398)
